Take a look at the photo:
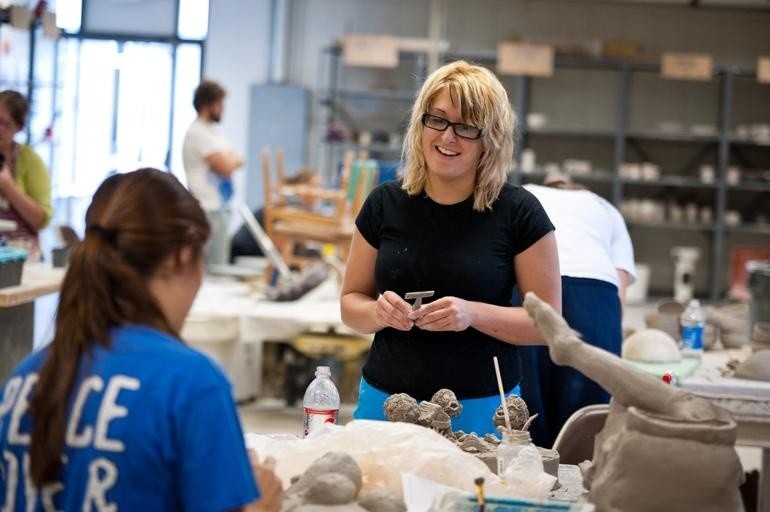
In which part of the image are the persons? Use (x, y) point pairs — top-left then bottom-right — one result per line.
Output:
(339, 56), (566, 441)
(0, 164), (287, 512)
(230, 169), (323, 265)
(509, 177), (640, 452)
(182, 79), (244, 267)
(0, 88), (54, 260)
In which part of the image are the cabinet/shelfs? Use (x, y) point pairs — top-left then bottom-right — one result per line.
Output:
(321, 40), (770, 302)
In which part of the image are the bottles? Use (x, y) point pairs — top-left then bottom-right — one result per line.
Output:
(301, 366), (340, 436)
(680, 299), (705, 356)
(495, 432), (540, 478)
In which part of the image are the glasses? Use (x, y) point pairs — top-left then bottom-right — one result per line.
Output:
(419, 111), (487, 142)
